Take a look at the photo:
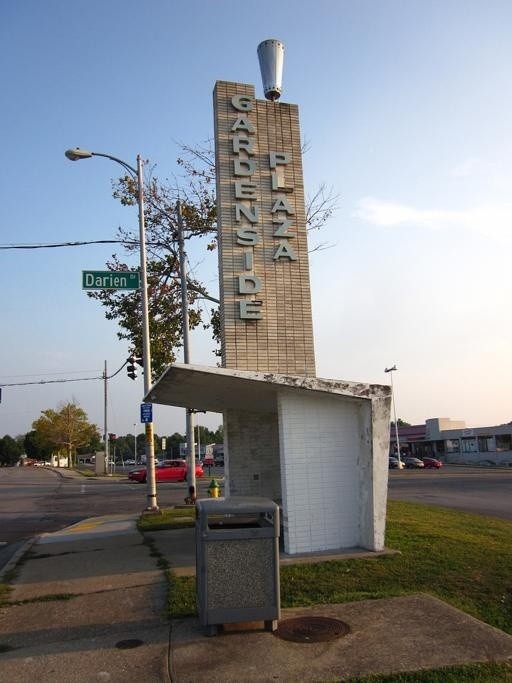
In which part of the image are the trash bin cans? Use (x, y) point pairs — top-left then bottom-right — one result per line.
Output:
(194, 498), (280, 636)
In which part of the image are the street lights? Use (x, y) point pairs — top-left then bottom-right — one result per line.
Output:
(61, 143), (162, 515)
(384, 363), (404, 470)
(133, 423), (138, 467)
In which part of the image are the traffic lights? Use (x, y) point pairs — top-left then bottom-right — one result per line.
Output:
(137, 352), (143, 368)
(126, 355), (138, 381)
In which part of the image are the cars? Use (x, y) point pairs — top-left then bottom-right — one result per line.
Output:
(21, 454), (204, 467)
(401, 455), (425, 469)
(419, 456), (443, 469)
(127, 459), (206, 484)
(389, 455), (407, 471)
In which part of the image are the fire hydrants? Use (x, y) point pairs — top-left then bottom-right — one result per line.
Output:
(206, 476), (223, 500)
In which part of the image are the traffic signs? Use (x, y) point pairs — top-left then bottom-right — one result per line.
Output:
(80, 268), (141, 291)
